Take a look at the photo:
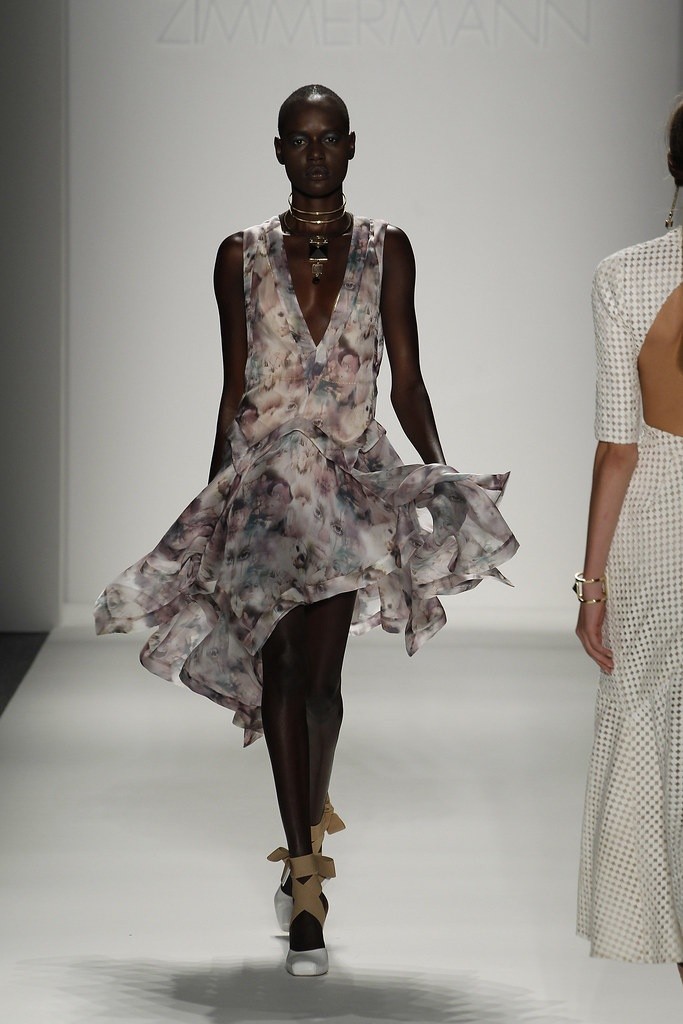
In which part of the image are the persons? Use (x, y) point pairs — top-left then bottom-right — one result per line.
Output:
(93, 84), (520, 979)
(573, 95), (683, 997)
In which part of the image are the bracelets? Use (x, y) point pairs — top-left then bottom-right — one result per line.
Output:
(572, 571), (607, 604)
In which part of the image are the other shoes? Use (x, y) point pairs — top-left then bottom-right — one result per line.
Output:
(274, 792), (345, 932)
(267, 846), (336, 976)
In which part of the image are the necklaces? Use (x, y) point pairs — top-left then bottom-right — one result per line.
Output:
(283, 192), (352, 285)
